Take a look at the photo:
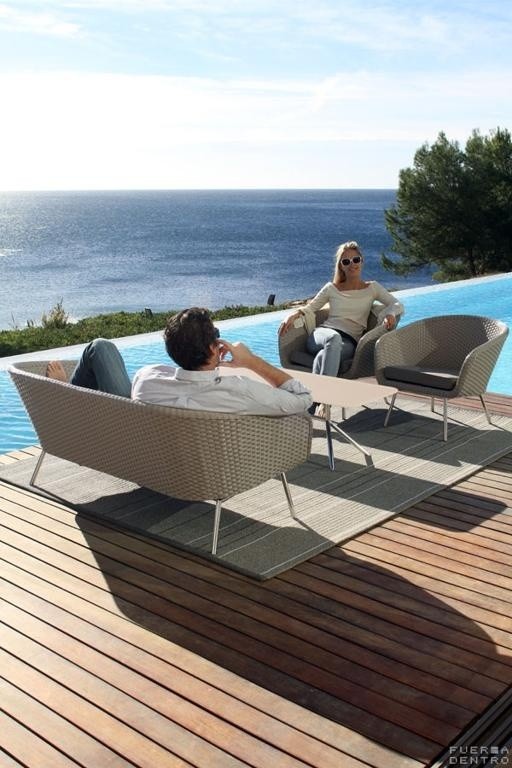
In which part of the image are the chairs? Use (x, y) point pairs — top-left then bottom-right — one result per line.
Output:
(277, 304), (401, 420)
(373, 315), (509, 442)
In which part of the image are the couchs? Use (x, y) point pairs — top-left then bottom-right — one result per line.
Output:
(6, 359), (313, 555)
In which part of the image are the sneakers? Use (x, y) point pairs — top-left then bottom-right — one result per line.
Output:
(318, 404), (330, 419)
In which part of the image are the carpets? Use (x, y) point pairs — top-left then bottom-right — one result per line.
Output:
(0, 396), (512, 582)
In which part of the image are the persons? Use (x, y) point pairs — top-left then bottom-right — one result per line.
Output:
(280, 241), (403, 421)
(46, 307), (313, 417)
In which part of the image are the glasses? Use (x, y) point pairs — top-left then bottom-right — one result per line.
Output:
(215, 328), (220, 338)
(341, 256), (362, 267)
